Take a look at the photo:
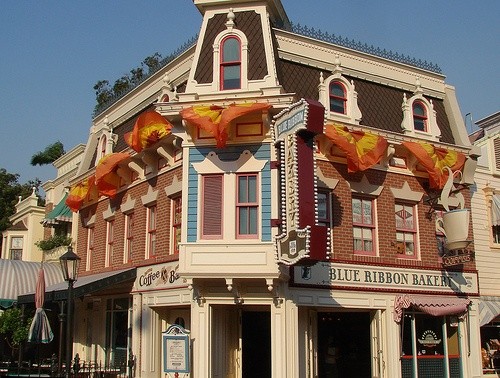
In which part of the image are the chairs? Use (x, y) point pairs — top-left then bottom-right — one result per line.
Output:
(1, 357), (124, 378)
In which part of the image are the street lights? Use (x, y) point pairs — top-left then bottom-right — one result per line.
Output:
(58, 244), (82, 378)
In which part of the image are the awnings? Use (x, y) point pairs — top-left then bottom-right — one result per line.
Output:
(39, 191), (72, 228)
(478, 296), (500, 326)
(394, 292), (472, 323)
(16, 266), (135, 304)
(491, 194), (500, 226)
(0, 258), (68, 308)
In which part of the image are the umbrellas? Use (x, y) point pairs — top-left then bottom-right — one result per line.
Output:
(27, 258), (54, 377)
